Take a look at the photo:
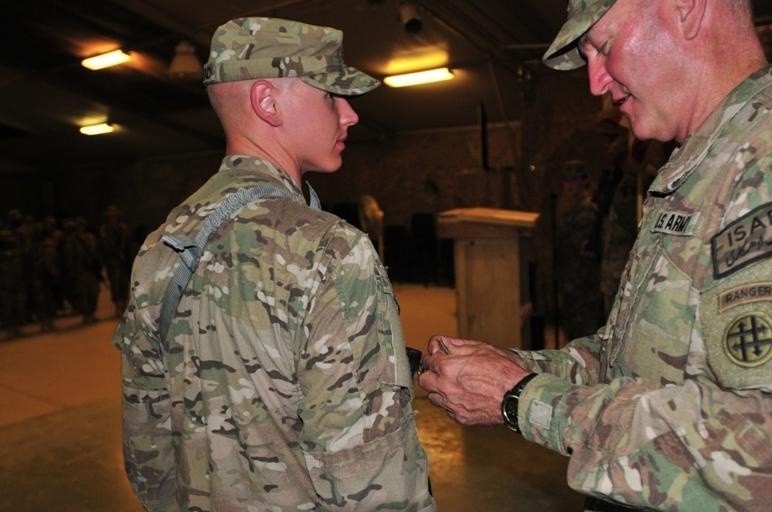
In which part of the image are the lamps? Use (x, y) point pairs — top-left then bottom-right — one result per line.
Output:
(382, 67), (456, 90)
(397, 2), (428, 36)
(77, 46), (132, 72)
(78, 115), (118, 136)
(165, 39), (203, 84)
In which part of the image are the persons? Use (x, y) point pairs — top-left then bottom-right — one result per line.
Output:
(551, 159), (605, 337)
(0, 204), (134, 340)
(108, 16), (437, 512)
(595, 108), (665, 319)
(417, 1), (770, 512)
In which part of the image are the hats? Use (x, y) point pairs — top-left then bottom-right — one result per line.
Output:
(558, 160), (590, 179)
(201, 15), (382, 97)
(543, 0), (618, 72)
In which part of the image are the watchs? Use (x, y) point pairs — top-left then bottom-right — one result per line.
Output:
(499, 372), (538, 434)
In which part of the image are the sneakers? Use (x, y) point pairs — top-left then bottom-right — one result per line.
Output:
(7, 325), (27, 339)
(83, 314), (99, 325)
(41, 320), (61, 333)
(116, 300), (128, 318)
